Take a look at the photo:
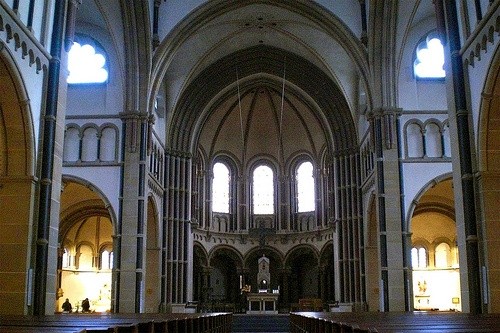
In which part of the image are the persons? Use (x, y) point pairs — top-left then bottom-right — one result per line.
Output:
(62, 299), (72, 313)
(82, 298), (90, 312)
(240, 284), (251, 310)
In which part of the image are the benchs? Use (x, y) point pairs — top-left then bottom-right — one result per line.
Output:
(290, 310), (500, 333)
(0, 311), (235, 333)
